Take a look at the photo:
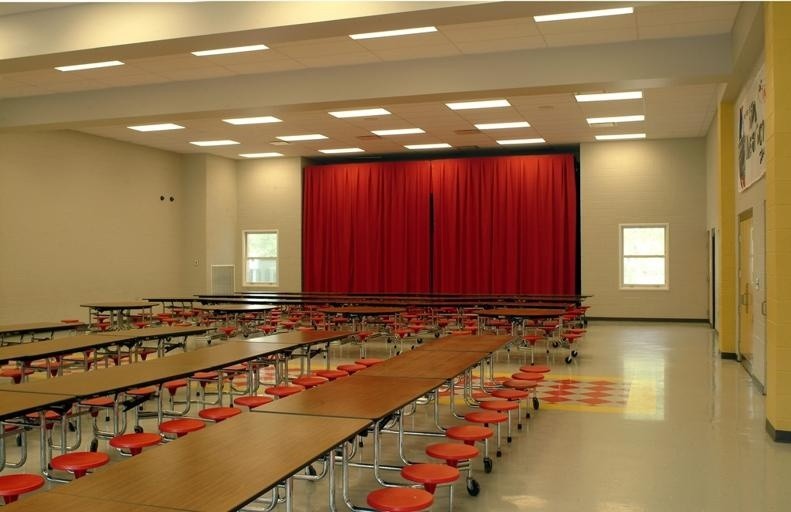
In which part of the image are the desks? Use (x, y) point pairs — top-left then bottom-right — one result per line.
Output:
(352, 350), (502, 420)
(319, 307), (407, 329)
(236, 290), (595, 299)
(193, 293), (587, 307)
(0, 342), (297, 458)
(97, 326), (218, 362)
(235, 331), (357, 387)
(0, 335), (137, 384)
(194, 305), (277, 331)
(248, 377), (466, 488)
(80, 302), (161, 331)
(143, 296), (575, 311)
(0, 389), (77, 486)
(419, 335), (514, 386)
(478, 308), (566, 349)
(0, 320), (84, 342)
(46, 411), (378, 512)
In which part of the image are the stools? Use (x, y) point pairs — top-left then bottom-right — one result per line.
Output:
(0, 367), (35, 383)
(410, 319), (426, 325)
(479, 402), (520, 443)
(0, 419), (28, 468)
(95, 323), (110, 331)
(1, 472), (45, 506)
(367, 487), (433, 512)
(37, 361), (73, 376)
(25, 410), (72, 469)
(357, 331), (370, 357)
(92, 316), (109, 322)
(581, 305), (592, 325)
(560, 316), (571, 328)
(200, 320), (216, 326)
(242, 359), (261, 391)
(441, 307), (458, 312)
(157, 378), (190, 419)
(312, 369), (350, 381)
(281, 322), (297, 332)
(159, 313), (173, 316)
(488, 320), (503, 335)
(540, 326), (557, 337)
(136, 322), (149, 328)
(265, 387), (304, 398)
(190, 310), (201, 318)
(191, 371), (219, 408)
(161, 317), (178, 327)
(565, 330), (588, 356)
(355, 358), (386, 368)
(311, 318), (324, 323)
(267, 319), (281, 326)
(444, 425), (494, 473)
(221, 363), (247, 410)
(511, 373), (544, 410)
(155, 315), (170, 321)
(159, 420), (206, 438)
(420, 313), (432, 317)
(402, 313), (418, 319)
(199, 407), (242, 422)
(435, 320), (447, 335)
(80, 397), (114, 451)
(520, 366), (550, 374)
(396, 330), (411, 355)
(401, 464), (461, 512)
(288, 317), (302, 322)
(220, 327), (236, 341)
(426, 443), (482, 496)
(461, 319), (477, 325)
(271, 312), (284, 316)
(383, 320), (395, 331)
(233, 396), (274, 410)
(292, 376), (330, 389)
(129, 314), (143, 321)
(463, 326), (477, 332)
(574, 308), (586, 329)
(124, 386), (157, 433)
(60, 319), (80, 323)
(109, 433), (162, 456)
(561, 334), (582, 363)
(503, 380), (537, 420)
(50, 452), (109, 479)
(259, 326), (274, 335)
(523, 335), (545, 367)
(407, 325), (428, 344)
(291, 312), (304, 317)
(465, 412), (509, 459)
(337, 364), (368, 376)
(171, 310), (183, 317)
(182, 312), (191, 320)
(492, 390), (528, 430)
(335, 318), (349, 330)
